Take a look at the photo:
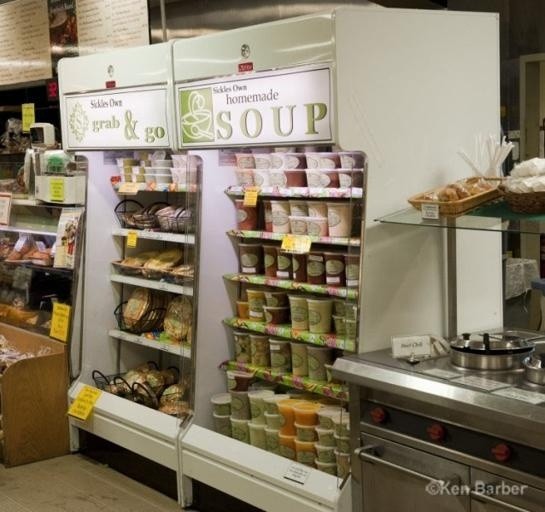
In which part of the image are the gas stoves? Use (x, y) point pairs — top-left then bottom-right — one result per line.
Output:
(330, 348), (544, 512)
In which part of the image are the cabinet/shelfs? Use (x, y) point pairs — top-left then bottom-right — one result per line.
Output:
(59, 9), (499, 512)
(0, 194), (84, 345)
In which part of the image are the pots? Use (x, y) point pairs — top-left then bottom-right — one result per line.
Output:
(449, 331), (535, 372)
(521, 352), (545, 386)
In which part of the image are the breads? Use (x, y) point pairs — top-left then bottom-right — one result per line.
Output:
(110, 363), (148, 382)
(157, 375), (191, 413)
(164, 297), (194, 343)
(122, 287), (162, 331)
(120, 251), (157, 266)
(145, 250), (183, 270)
(29, 248), (54, 258)
(9, 242), (34, 262)
(130, 367), (179, 387)
(420, 178), (496, 200)
(1, 244), (12, 256)
